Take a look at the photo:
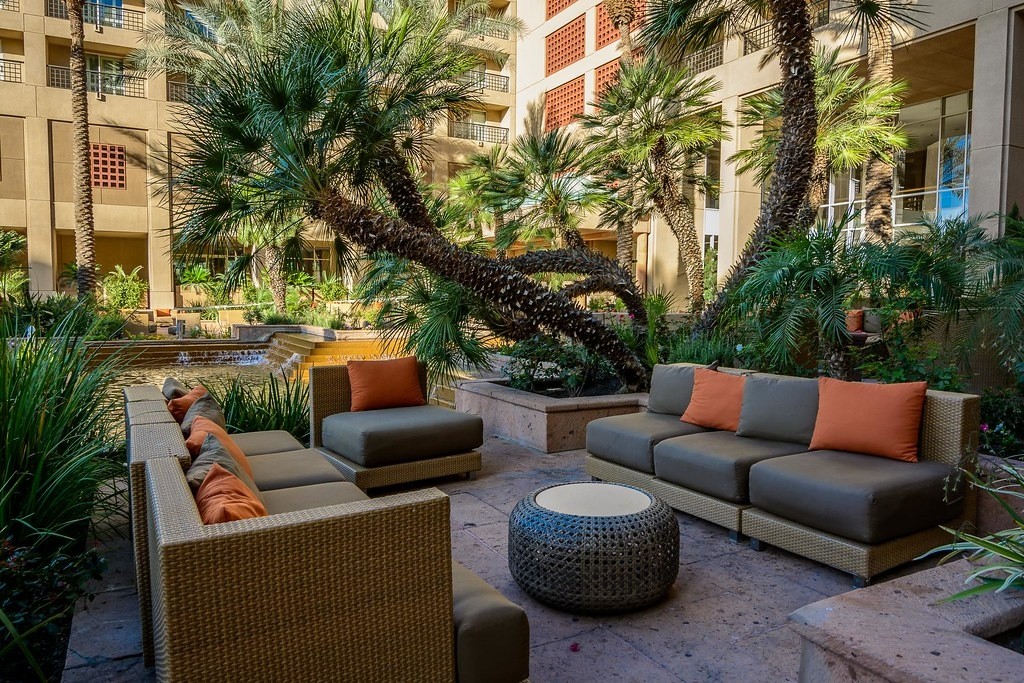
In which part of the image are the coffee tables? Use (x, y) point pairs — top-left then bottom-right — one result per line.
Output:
(508, 480), (681, 614)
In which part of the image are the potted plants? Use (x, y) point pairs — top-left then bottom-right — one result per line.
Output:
(863, 288), (883, 332)
(845, 290), (864, 334)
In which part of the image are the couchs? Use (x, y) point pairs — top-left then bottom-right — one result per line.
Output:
(583, 363), (979, 589)
(308, 360), (484, 490)
(120, 383), (451, 683)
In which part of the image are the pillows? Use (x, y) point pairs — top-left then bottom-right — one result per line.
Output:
(679, 367), (745, 431)
(807, 376), (927, 462)
(347, 356), (428, 413)
(733, 373), (818, 445)
(647, 361), (718, 416)
